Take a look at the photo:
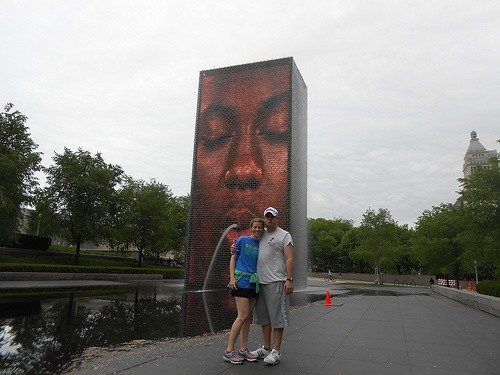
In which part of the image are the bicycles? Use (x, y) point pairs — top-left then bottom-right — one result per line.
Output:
(324, 274), (337, 282)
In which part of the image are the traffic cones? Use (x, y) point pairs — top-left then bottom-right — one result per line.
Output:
(323, 289), (333, 306)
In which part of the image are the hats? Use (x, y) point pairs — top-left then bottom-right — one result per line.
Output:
(264, 207), (278, 216)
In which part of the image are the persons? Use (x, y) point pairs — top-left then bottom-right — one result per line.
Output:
(328, 270), (333, 281)
(429, 277), (434, 285)
(222, 216), (265, 365)
(230, 207), (295, 365)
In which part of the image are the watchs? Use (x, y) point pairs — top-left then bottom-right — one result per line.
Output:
(287, 278), (294, 283)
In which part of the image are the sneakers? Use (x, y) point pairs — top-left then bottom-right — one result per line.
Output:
(264, 349), (281, 364)
(223, 350), (242, 364)
(252, 345), (272, 358)
(239, 348), (257, 361)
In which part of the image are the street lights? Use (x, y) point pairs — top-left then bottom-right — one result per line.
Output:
(474, 260), (479, 285)
